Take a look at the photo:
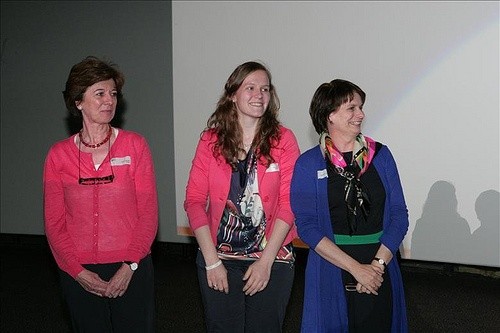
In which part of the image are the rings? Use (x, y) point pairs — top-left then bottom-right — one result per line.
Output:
(213, 284), (218, 288)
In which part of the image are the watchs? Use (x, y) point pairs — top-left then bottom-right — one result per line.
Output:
(372, 257), (387, 268)
(123, 261), (138, 271)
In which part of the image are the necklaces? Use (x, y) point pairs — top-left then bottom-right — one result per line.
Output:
(79, 124), (112, 148)
(239, 141), (252, 149)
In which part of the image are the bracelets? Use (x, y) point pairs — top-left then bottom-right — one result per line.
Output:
(205, 260), (222, 270)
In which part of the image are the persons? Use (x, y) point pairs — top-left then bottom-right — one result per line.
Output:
(184, 62), (301, 333)
(43, 57), (158, 333)
(289, 79), (409, 333)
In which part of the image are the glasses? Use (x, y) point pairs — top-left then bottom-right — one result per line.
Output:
(79, 175), (114, 185)
